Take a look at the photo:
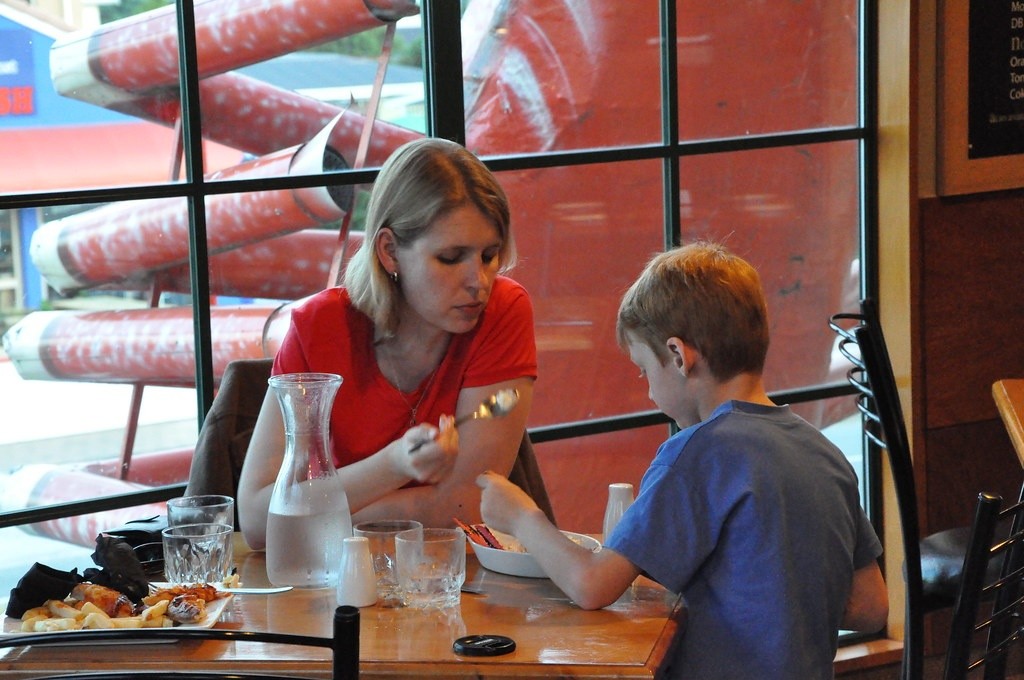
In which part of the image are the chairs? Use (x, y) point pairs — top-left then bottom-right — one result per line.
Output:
(809, 295), (1023, 680)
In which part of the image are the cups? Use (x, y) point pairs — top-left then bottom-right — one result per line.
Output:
(161, 523), (234, 585)
(166, 495), (234, 536)
(353, 520), (423, 599)
(394, 528), (466, 608)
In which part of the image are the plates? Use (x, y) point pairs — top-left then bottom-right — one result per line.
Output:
(0, 582), (245, 643)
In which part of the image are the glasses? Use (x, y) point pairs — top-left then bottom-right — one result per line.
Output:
(131, 539), (191, 574)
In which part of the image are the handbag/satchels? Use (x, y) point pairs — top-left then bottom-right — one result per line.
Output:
(95, 515), (169, 574)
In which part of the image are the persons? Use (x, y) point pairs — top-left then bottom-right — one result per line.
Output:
(474, 229), (890, 680)
(236, 136), (540, 553)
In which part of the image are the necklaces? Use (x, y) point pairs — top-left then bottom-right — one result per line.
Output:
(379, 341), (447, 418)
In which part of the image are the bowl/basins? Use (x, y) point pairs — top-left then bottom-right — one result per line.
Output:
(467, 523), (603, 578)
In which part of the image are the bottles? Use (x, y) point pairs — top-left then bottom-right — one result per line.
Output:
(337, 537), (376, 606)
(264, 374), (355, 591)
(603, 483), (634, 544)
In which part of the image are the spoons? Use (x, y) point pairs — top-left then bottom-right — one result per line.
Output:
(408, 388), (520, 457)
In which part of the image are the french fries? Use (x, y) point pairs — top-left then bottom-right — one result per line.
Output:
(19, 597), (170, 631)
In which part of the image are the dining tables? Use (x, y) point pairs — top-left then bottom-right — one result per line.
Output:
(0, 535), (686, 680)
